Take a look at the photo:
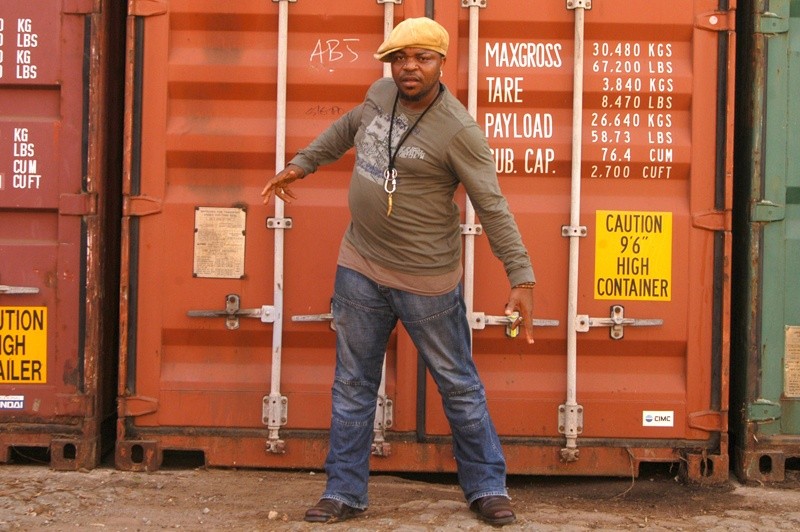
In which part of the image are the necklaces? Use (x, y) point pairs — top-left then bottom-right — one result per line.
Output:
(384, 83), (442, 216)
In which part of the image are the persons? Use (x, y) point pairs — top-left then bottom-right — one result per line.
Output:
(260, 17), (537, 525)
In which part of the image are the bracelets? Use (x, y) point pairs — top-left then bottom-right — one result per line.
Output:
(514, 283), (534, 289)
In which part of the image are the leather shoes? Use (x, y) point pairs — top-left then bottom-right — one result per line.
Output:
(470, 495), (515, 524)
(305, 498), (366, 522)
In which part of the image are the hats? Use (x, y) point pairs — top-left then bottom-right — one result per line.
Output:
(373, 17), (449, 62)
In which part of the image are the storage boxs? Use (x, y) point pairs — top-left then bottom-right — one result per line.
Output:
(1, 0), (799, 486)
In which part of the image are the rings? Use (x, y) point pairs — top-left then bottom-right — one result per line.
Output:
(518, 316), (523, 320)
